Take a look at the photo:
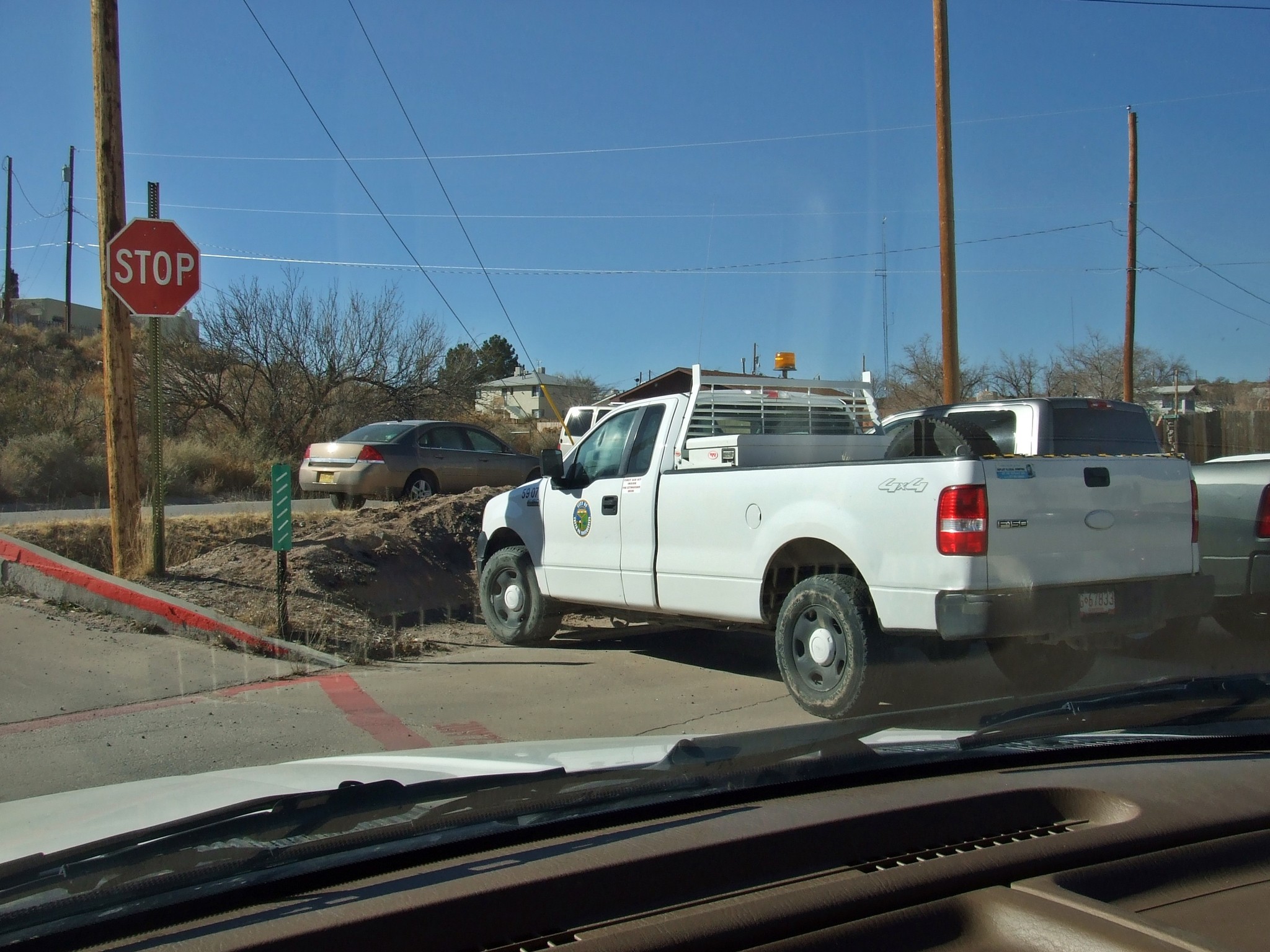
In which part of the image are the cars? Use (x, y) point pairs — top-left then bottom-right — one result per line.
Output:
(299, 420), (542, 512)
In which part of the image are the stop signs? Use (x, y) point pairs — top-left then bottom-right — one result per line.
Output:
(106, 217), (201, 318)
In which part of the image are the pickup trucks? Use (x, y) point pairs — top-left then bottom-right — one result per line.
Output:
(863, 394), (1270, 639)
(472, 353), (1202, 721)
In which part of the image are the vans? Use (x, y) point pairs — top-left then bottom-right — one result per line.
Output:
(557, 406), (618, 459)
